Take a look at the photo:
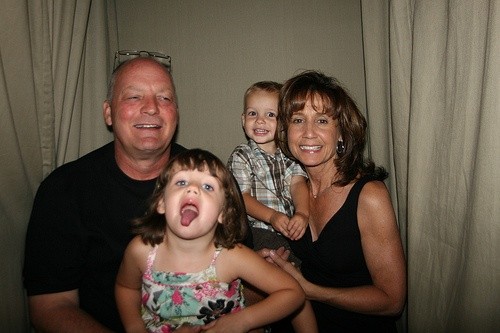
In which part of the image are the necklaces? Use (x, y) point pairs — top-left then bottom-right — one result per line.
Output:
(306, 171), (343, 200)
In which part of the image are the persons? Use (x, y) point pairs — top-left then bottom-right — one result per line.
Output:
(223, 80), (318, 333)
(255, 69), (407, 333)
(21, 49), (255, 333)
(114, 149), (305, 333)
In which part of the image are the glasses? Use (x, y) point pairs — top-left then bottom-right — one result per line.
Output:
(112, 48), (172, 75)
